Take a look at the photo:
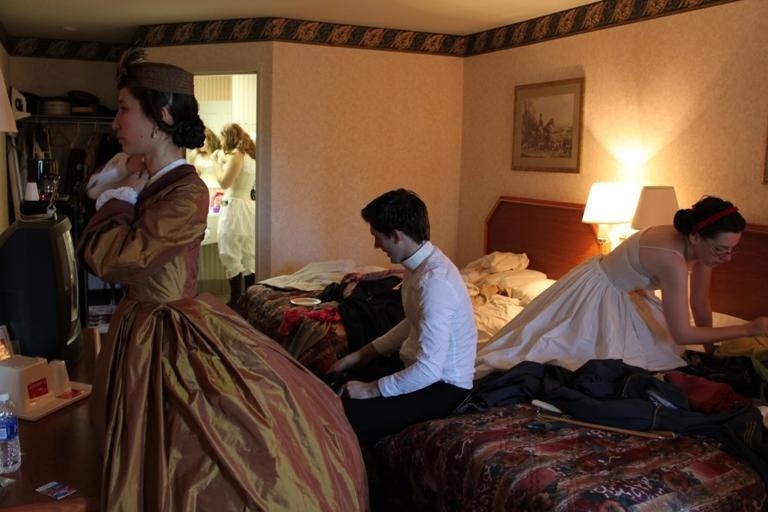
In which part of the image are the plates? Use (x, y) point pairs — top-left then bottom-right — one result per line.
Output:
(290, 298), (321, 306)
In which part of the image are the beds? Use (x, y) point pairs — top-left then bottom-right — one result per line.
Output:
(376, 225), (768, 512)
(235, 195), (606, 391)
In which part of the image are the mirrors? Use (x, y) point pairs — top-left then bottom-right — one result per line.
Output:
(183, 75), (231, 245)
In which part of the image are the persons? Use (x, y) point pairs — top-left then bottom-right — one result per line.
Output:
(190, 127), (222, 199)
(323, 189), (478, 449)
(81, 60), (372, 512)
(213, 123), (257, 308)
(474, 196), (763, 383)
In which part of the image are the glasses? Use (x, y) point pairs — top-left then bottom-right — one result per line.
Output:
(698, 230), (743, 259)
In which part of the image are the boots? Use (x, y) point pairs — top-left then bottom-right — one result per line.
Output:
(244, 272), (256, 291)
(226, 272), (243, 308)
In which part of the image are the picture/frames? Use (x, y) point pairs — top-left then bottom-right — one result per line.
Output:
(511, 77), (585, 174)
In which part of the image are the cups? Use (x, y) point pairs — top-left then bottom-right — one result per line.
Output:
(46, 359), (70, 393)
(25, 183), (39, 201)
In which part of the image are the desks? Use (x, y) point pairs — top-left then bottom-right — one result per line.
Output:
(0, 327), (104, 512)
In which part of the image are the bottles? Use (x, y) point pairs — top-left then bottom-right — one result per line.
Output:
(0, 392), (21, 474)
(81, 326), (100, 358)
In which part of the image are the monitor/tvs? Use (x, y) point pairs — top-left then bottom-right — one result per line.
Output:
(1, 215), (83, 360)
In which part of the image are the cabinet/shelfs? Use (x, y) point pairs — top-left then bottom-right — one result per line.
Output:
(14, 116), (132, 307)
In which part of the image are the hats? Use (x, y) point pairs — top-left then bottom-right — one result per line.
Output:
(116, 60), (196, 96)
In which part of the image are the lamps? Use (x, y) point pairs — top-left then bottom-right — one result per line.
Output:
(582, 182), (634, 255)
(632, 185), (680, 231)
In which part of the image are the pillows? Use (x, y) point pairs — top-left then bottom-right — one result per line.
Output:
(512, 278), (556, 307)
(479, 264), (547, 297)
